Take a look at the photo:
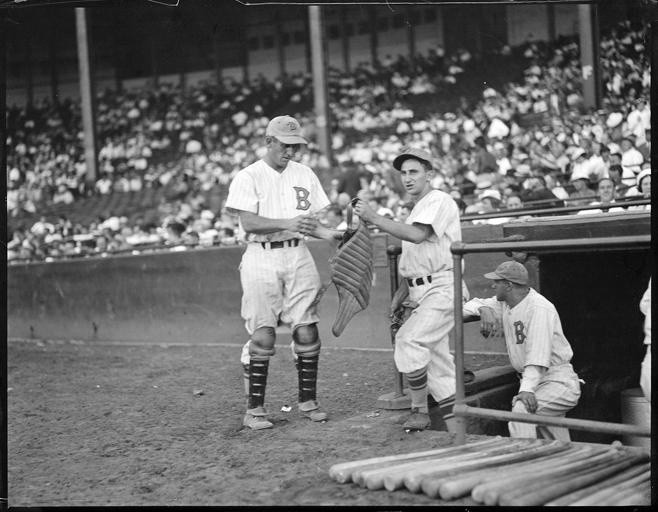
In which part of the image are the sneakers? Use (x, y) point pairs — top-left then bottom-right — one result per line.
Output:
(401, 411), (431, 433)
(296, 400), (328, 422)
(242, 407), (273, 431)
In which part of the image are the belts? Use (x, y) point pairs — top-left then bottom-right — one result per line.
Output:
(262, 238), (300, 249)
(408, 275), (432, 286)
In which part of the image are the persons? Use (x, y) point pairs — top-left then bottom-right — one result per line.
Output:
(353, 147), (472, 434)
(223, 114), (347, 432)
(462, 259), (588, 442)
(2, 19), (651, 260)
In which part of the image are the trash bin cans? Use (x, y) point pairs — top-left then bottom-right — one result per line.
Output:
(621, 388), (652, 446)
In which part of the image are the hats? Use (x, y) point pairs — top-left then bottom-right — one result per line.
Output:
(393, 148), (433, 169)
(483, 260), (529, 286)
(265, 116), (308, 145)
